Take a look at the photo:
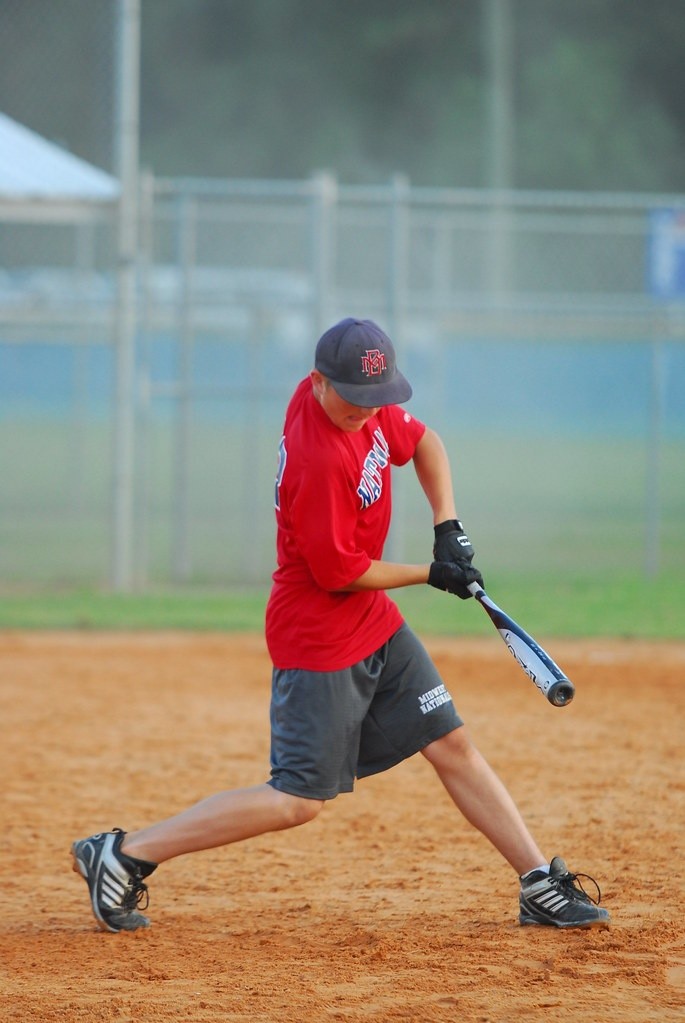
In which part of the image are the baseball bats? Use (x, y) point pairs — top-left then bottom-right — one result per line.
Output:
(467, 581), (576, 707)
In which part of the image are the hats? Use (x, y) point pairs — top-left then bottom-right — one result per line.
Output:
(314, 317), (413, 407)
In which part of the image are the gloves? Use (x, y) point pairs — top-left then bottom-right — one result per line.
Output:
(428, 562), (484, 600)
(433, 519), (475, 564)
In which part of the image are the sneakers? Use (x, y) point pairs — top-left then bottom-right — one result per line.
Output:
(518, 856), (609, 927)
(72, 828), (158, 932)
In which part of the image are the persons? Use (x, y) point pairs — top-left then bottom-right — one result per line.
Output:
(70, 316), (611, 935)
